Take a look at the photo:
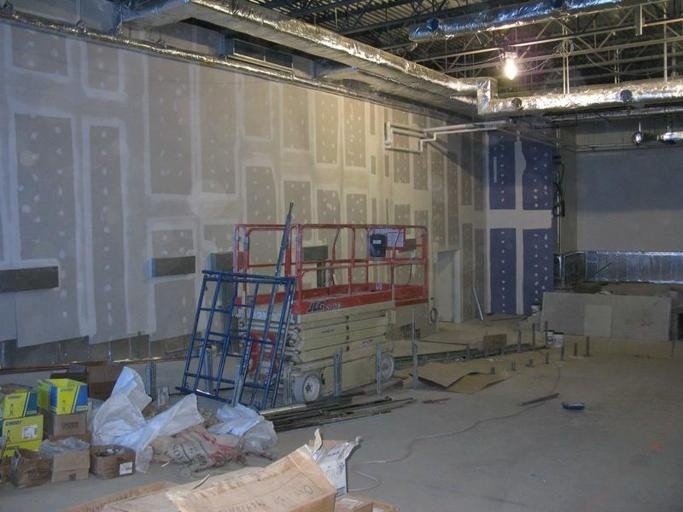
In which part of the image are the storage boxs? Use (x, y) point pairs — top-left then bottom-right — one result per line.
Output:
(0, 377), (135, 487)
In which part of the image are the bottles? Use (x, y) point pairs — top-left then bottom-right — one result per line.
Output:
(552, 332), (564, 349)
(544, 330), (554, 347)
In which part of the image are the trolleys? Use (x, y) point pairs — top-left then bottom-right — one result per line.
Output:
(234, 283), (438, 402)
